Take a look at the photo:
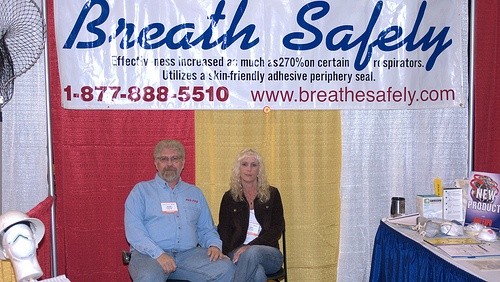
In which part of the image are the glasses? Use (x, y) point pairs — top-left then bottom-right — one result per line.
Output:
(156, 156), (182, 162)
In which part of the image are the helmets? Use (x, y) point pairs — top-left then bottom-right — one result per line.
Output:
(1, 212), (45, 260)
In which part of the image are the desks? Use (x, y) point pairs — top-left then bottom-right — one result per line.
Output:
(368, 213), (500, 282)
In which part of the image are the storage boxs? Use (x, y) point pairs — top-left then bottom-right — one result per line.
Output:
(416, 188), (463, 222)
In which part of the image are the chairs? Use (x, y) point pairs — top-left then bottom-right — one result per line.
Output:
(122, 218), (287, 282)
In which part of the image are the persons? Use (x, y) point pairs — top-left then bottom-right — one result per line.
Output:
(217, 147), (284, 282)
(124, 139), (235, 282)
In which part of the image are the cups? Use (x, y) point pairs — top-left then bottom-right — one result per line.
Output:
(391, 197), (405, 218)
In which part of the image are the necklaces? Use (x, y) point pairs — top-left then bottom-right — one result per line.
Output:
(246, 197), (256, 206)
